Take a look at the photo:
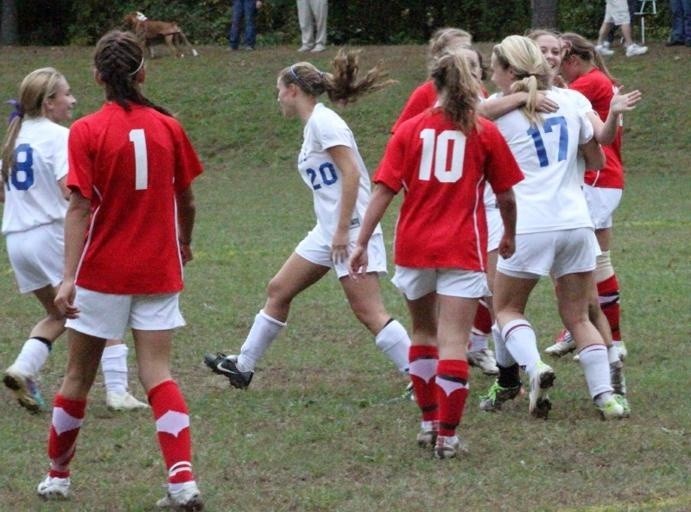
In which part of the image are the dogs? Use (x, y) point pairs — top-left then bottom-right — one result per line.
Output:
(123, 9), (198, 60)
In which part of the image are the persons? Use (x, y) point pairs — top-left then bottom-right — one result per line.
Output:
(592, 0), (690, 60)
(388, 27), (643, 420)
(291, 1), (333, 54)
(2, 64), (154, 418)
(200, 43), (429, 402)
(35, 23), (212, 506)
(346, 44), (525, 459)
(223, 1), (263, 50)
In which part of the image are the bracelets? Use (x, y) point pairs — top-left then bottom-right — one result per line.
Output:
(177, 238), (192, 247)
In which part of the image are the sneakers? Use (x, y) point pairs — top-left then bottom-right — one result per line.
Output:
(37, 475), (71, 498)
(310, 44), (326, 52)
(2, 364), (45, 415)
(105, 390), (152, 412)
(156, 481), (204, 511)
(297, 45), (310, 53)
(203, 352), (255, 391)
(415, 328), (631, 460)
(594, 44), (614, 56)
(626, 43), (648, 57)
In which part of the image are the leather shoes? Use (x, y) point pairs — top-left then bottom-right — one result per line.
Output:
(666, 40), (685, 47)
